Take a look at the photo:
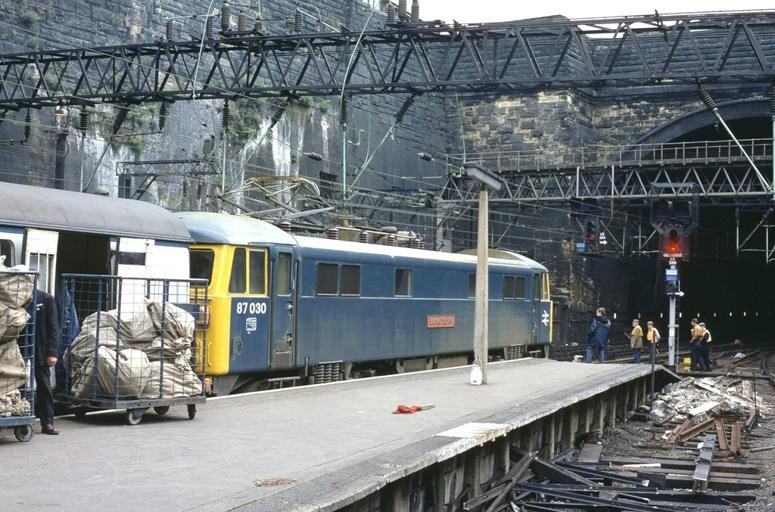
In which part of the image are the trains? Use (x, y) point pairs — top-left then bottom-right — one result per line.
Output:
(0, 180), (553, 423)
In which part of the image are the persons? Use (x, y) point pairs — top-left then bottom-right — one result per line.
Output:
(646, 320), (660, 364)
(16, 268), (61, 434)
(698, 322), (713, 371)
(631, 318), (643, 363)
(688, 318), (707, 372)
(585, 307), (612, 363)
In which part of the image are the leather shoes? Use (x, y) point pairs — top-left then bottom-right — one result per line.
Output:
(41, 424), (59, 434)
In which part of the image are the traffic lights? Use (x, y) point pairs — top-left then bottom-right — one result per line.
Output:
(664, 229), (683, 258)
(584, 220), (599, 255)
